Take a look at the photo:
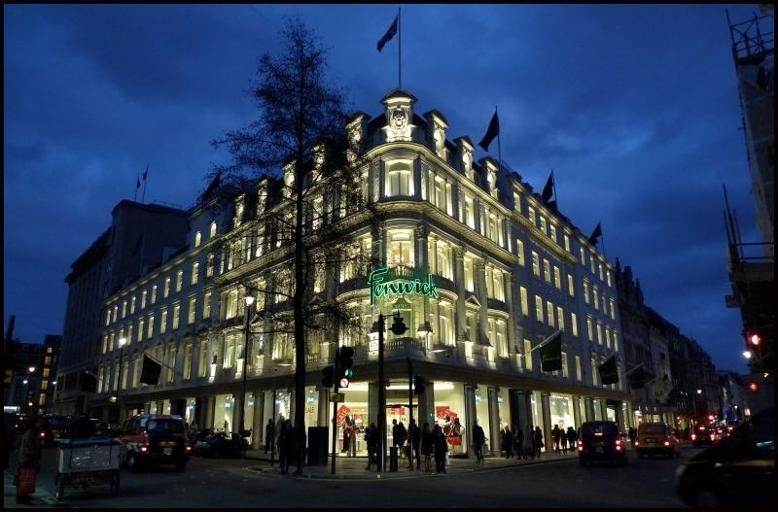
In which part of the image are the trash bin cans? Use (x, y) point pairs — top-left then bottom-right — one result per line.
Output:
(286, 435), (301, 474)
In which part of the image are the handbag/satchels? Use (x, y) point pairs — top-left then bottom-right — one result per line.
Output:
(17, 468), (36, 493)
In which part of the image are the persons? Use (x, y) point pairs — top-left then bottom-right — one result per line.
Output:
(265, 419), (305, 475)
(17, 415), (41, 503)
(343, 416), (580, 474)
(75, 413), (87, 440)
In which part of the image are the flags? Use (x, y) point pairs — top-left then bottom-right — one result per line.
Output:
(136, 178), (142, 188)
(542, 175), (553, 202)
(378, 14), (399, 51)
(142, 169), (147, 179)
(598, 357), (619, 385)
(140, 355), (161, 385)
(479, 110), (498, 152)
(592, 223), (601, 238)
(539, 333), (562, 372)
(625, 366), (644, 390)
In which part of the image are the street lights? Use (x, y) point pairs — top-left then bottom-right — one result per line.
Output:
(238, 288), (256, 439)
(377, 310), (409, 472)
(111, 336), (127, 432)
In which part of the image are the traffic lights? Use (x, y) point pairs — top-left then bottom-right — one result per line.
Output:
(340, 345), (354, 379)
(321, 365), (334, 388)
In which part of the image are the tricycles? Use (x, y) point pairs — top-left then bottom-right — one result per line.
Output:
(54, 431), (121, 502)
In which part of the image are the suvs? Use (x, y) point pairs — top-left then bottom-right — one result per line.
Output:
(118, 413), (191, 466)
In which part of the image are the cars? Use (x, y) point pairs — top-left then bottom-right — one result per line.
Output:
(677, 410), (777, 512)
(689, 420), (738, 446)
(15, 412), (107, 457)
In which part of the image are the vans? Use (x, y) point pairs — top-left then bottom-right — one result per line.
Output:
(578, 420), (623, 464)
(634, 422), (676, 458)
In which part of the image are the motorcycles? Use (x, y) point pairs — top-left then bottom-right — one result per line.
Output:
(196, 427), (252, 455)
